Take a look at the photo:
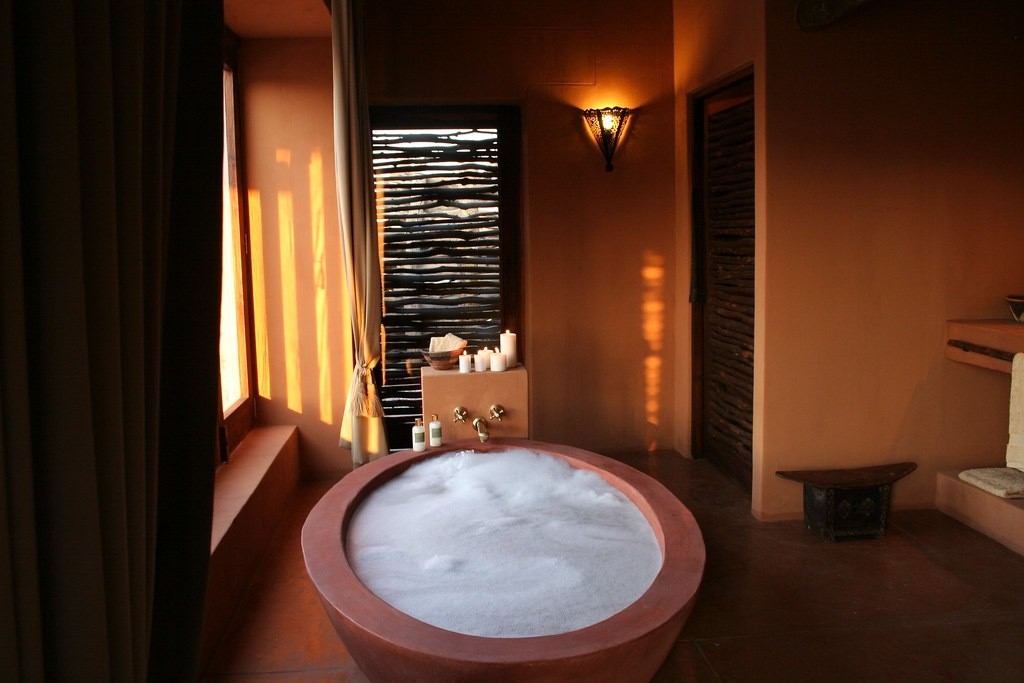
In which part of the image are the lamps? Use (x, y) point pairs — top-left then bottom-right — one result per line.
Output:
(578, 106), (636, 173)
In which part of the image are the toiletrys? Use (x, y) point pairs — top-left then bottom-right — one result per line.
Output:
(412, 418), (426, 452)
(428, 414), (442, 447)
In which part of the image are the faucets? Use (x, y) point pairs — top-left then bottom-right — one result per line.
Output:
(472, 417), (490, 443)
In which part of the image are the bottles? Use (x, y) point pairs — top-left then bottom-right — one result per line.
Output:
(429, 414), (443, 446)
(412, 417), (425, 452)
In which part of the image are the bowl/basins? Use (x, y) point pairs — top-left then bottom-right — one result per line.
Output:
(1004, 294), (1024, 323)
(420, 347), (462, 371)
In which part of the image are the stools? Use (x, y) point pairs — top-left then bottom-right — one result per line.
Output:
(774, 461), (918, 544)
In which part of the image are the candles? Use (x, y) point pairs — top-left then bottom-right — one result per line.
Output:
(473, 350), (486, 371)
(477, 346), (494, 368)
(499, 329), (517, 368)
(490, 347), (506, 372)
(458, 349), (472, 373)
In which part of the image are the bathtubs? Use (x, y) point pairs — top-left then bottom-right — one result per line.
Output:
(299, 435), (709, 683)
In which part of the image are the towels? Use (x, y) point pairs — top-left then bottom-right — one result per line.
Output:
(957, 466), (1024, 499)
(1005, 352), (1024, 473)
(428, 331), (468, 352)
